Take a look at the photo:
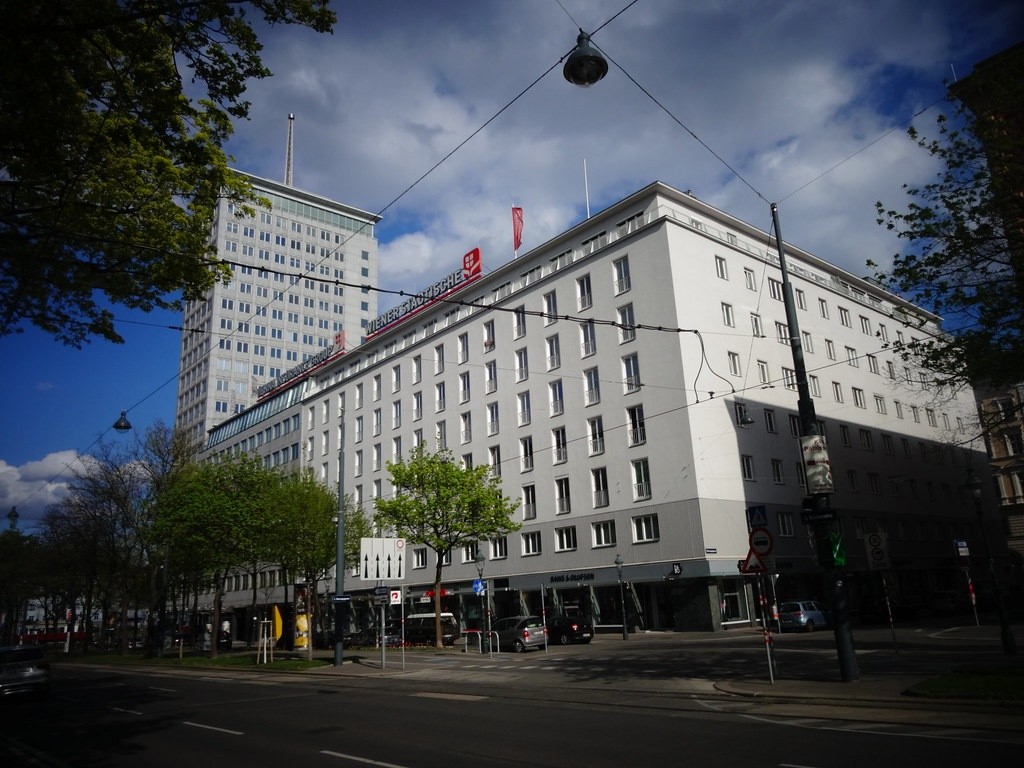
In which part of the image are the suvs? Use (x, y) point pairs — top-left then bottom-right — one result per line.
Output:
(778, 600), (827, 633)
(354, 627), (402, 648)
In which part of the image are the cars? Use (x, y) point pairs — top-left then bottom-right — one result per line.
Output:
(546, 616), (595, 645)
(485, 615), (549, 654)
(0, 662), (51, 704)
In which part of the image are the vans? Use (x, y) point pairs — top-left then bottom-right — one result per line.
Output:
(404, 613), (462, 645)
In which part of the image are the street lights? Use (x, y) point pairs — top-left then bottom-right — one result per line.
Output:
(475, 549), (489, 654)
(614, 552), (629, 640)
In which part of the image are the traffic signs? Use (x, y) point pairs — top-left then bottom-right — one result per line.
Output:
(955, 540), (968, 548)
(373, 586), (387, 596)
